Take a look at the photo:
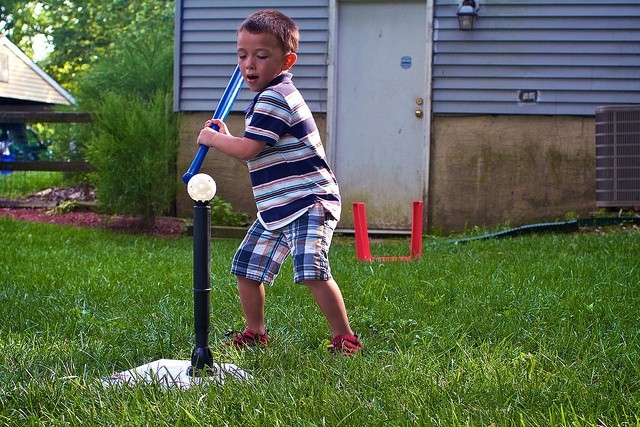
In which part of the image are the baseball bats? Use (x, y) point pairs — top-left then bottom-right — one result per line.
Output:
(183, 64), (245, 184)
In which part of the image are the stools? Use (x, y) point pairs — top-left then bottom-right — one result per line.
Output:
(351, 201), (424, 263)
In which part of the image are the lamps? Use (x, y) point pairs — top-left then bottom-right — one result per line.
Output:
(455, 1), (478, 30)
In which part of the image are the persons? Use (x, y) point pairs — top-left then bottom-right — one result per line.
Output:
(197, 8), (362, 355)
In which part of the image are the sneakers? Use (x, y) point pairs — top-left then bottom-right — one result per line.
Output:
(328, 335), (360, 358)
(225, 327), (270, 348)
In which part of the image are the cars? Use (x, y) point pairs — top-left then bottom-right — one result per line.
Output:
(0, 123), (52, 161)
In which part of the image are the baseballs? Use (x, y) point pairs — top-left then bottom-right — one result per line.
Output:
(187, 174), (217, 201)
